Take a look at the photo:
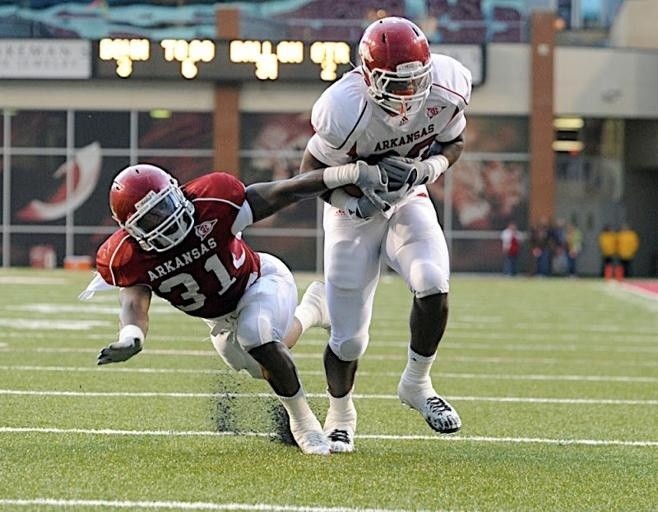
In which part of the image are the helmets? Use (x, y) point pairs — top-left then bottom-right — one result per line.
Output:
(109, 164), (197, 254)
(358, 16), (434, 130)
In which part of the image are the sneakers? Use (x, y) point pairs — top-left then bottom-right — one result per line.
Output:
(398, 377), (462, 433)
(289, 411), (331, 455)
(304, 278), (333, 340)
(322, 398), (357, 452)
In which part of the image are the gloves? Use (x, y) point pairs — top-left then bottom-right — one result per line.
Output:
(353, 155), (431, 222)
(96, 336), (143, 365)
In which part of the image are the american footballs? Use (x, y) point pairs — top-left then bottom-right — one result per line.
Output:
(342, 166), (403, 197)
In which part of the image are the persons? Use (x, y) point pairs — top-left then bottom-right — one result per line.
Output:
(96, 160), (403, 456)
(300, 17), (472, 454)
(530, 215), (551, 277)
(500, 222), (521, 276)
(565, 222), (583, 279)
(598, 224), (616, 278)
(550, 216), (568, 276)
(615, 222), (640, 277)
